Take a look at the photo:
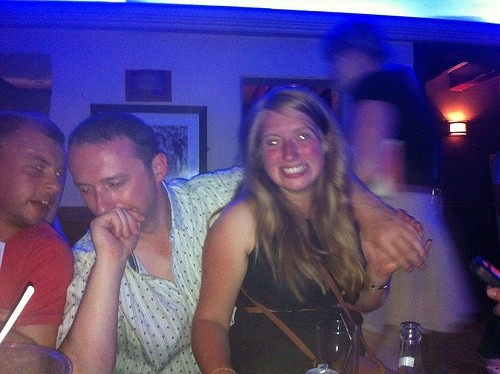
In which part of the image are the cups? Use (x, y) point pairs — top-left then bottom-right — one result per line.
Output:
(0, 342), (73, 374)
(315, 318), (354, 374)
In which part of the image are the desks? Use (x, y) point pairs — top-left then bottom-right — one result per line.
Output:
(359, 189), (478, 338)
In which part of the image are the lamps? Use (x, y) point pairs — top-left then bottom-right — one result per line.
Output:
(446, 110), (469, 137)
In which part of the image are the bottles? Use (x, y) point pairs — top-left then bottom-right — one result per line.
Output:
(396, 320), (424, 374)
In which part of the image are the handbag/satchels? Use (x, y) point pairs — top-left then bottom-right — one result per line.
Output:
(329, 350), (396, 374)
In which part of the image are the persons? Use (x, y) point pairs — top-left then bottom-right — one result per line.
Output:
(55, 111), (428, 374)
(191, 85), (433, 374)
(0, 111), (73, 374)
(321, 30), (500, 322)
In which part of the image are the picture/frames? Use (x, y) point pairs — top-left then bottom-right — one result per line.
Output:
(92, 102), (208, 176)
(123, 70), (172, 104)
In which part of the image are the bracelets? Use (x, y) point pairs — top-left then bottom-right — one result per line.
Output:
(368, 280), (390, 289)
